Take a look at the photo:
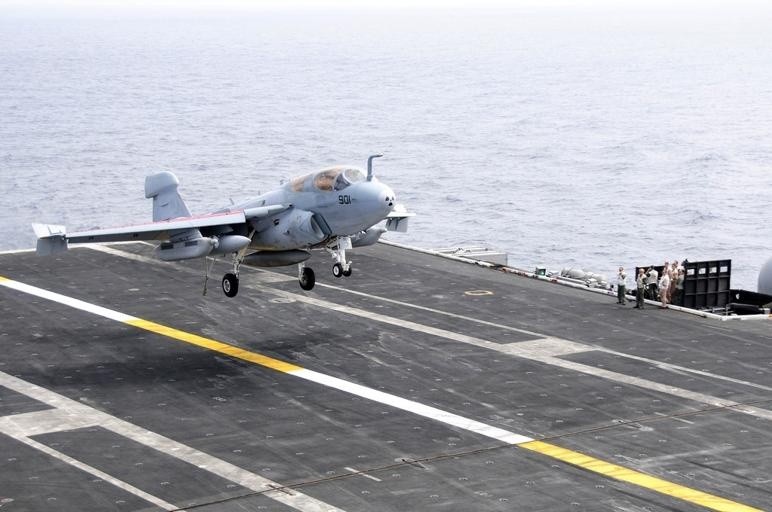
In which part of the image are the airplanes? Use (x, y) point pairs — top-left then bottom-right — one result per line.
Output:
(30, 152), (417, 298)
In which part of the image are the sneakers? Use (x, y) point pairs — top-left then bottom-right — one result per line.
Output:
(658, 305), (667, 309)
(632, 305), (645, 310)
(616, 301), (626, 306)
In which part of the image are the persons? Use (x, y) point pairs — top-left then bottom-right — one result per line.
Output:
(616, 259), (685, 310)
